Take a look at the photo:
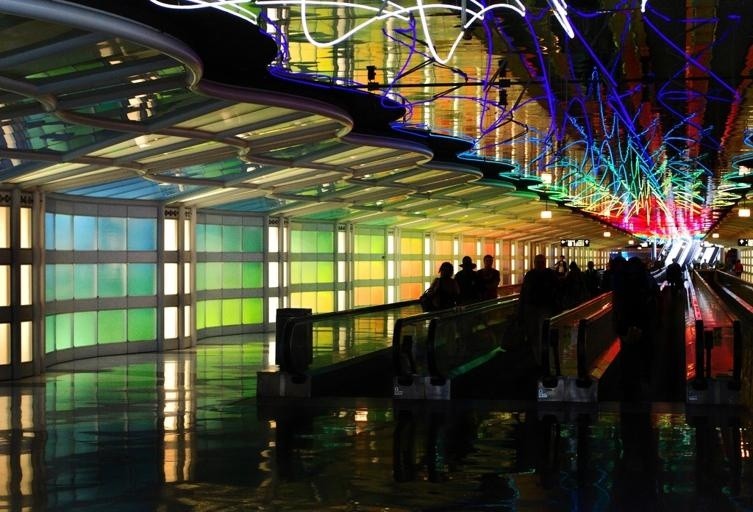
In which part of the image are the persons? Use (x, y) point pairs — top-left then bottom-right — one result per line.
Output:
(520, 256), (661, 368)
(667, 258), (682, 287)
(701, 256), (743, 277)
(420, 255), (500, 312)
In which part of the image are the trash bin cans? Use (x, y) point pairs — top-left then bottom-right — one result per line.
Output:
(275, 308), (312, 365)
